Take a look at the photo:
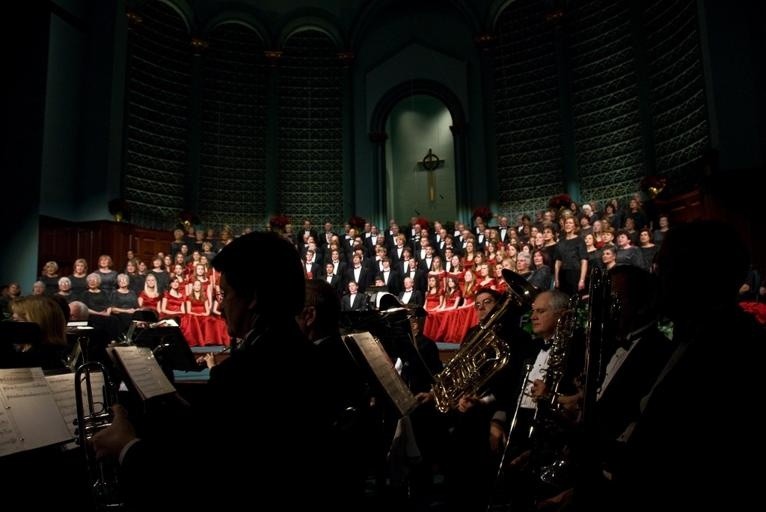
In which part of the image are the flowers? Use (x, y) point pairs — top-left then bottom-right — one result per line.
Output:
(548, 195), (571, 210)
(269, 215), (292, 229)
(473, 206), (493, 221)
(174, 210), (198, 227)
(348, 216), (366, 227)
(640, 173), (668, 193)
(108, 198), (132, 222)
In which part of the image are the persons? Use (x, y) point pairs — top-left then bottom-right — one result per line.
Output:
(1, 191), (765, 511)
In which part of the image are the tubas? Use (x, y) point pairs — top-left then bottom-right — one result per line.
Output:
(429, 269), (534, 414)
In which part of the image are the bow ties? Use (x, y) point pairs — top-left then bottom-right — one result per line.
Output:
(305, 229), (510, 295)
(618, 333), (638, 352)
(540, 340), (555, 352)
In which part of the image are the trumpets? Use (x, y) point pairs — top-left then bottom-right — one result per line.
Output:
(72, 335), (127, 509)
(535, 294), (588, 437)
(580, 259), (621, 435)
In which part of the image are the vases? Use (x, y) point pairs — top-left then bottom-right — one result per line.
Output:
(113, 213), (123, 222)
(353, 226), (361, 235)
(273, 228), (285, 236)
(649, 186), (660, 200)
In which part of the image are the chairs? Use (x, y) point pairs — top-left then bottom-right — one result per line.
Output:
(362, 398), (439, 511)
